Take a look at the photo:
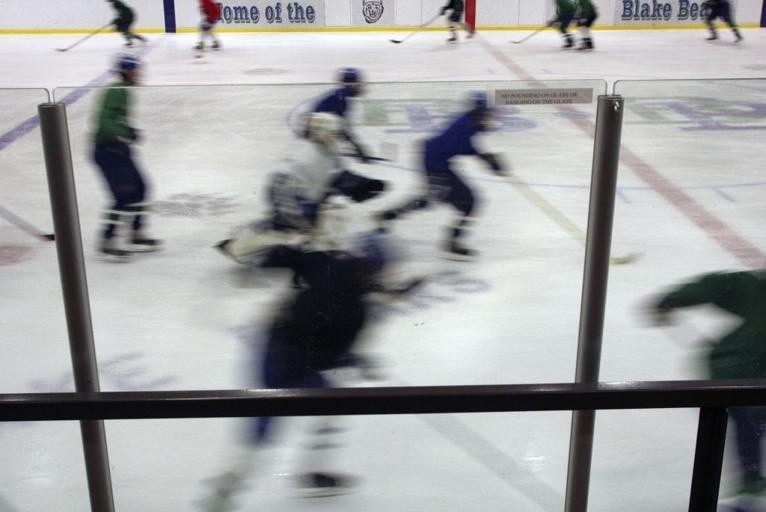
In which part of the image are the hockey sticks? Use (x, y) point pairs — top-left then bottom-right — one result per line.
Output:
(390, 13), (442, 43)
(54, 21), (117, 52)
(228, 266), (421, 296)
(0, 205), (58, 240)
(509, 175), (645, 264)
(509, 21), (555, 44)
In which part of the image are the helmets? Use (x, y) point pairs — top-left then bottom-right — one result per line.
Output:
(308, 66), (365, 138)
(111, 52), (144, 73)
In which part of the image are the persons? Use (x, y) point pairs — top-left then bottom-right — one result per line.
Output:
(192, 0), (225, 50)
(701, 1), (746, 45)
(548, 1), (577, 50)
(370, 91), (507, 256)
(636, 268), (766, 511)
(88, 54), (167, 259)
(202, 229), (413, 512)
(291, 66), (376, 166)
(441, 1), (476, 45)
(217, 116), (389, 274)
(571, 1), (599, 52)
(106, 0), (146, 49)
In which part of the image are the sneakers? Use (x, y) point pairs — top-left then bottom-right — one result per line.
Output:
(100, 225), (159, 255)
(705, 33), (742, 42)
(562, 40), (593, 51)
